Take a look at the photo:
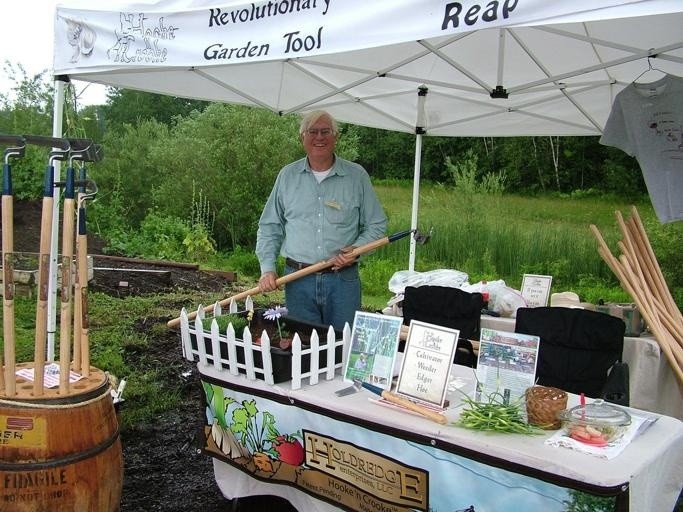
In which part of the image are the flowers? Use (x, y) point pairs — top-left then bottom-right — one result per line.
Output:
(260, 304), (288, 346)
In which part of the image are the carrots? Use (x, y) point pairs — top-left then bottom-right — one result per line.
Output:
(254, 451), (273, 472)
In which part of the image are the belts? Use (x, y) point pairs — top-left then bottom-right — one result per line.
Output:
(284, 256), (360, 275)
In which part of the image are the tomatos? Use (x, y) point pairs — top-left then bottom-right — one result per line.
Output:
(272, 435), (305, 466)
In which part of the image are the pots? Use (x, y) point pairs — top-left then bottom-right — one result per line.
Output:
(594, 303), (647, 337)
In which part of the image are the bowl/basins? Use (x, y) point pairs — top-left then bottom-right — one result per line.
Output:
(555, 401), (633, 448)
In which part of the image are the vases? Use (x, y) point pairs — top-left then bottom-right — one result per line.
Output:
(188, 306), (350, 383)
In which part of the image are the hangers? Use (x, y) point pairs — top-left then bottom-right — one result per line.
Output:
(632, 47), (670, 89)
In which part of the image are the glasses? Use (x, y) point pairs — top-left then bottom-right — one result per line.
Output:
(307, 128), (333, 137)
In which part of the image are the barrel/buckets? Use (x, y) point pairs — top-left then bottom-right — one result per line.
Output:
(0, 361), (122, 512)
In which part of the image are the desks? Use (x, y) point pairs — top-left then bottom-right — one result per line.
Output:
(193, 350), (683, 511)
(479, 313), (683, 418)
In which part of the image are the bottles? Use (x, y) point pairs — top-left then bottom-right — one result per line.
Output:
(482, 278), (489, 311)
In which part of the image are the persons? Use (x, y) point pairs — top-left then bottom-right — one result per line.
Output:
(253, 109), (388, 333)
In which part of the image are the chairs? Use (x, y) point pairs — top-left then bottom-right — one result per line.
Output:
(402, 285), (483, 363)
(513, 306), (630, 404)
